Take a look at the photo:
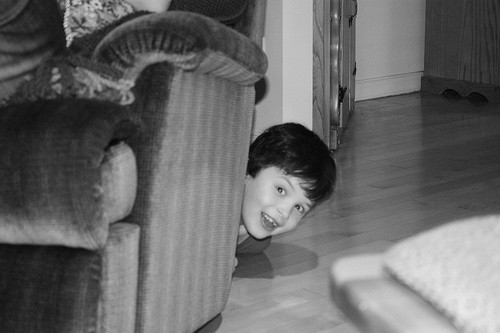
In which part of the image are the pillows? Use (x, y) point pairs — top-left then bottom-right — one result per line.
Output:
(381, 215), (500, 333)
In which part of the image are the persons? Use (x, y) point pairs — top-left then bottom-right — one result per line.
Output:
(226, 121), (339, 274)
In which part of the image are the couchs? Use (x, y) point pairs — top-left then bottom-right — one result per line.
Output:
(1, 0), (268, 333)
